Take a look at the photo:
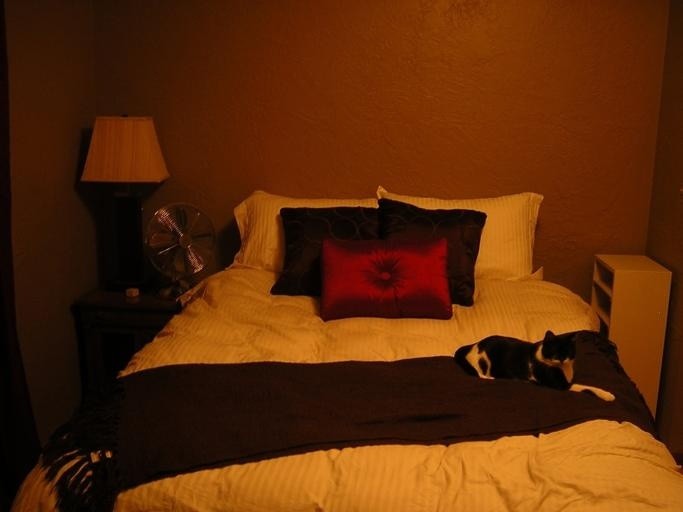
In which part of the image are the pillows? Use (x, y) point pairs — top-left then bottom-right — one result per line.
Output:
(268, 204), (379, 297)
(379, 196), (488, 309)
(230, 189), (378, 273)
(317, 236), (454, 324)
(375, 186), (544, 281)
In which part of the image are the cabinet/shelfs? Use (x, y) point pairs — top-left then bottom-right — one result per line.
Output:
(14, 267), (683, 511)
(590, 254), (673, 422)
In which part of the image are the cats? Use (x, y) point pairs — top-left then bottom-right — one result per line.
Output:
(453, 330), (616, 401)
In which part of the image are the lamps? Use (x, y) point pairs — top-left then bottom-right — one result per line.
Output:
(78, 113), (172, 294)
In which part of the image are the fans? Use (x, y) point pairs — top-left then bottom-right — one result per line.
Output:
(143, 201), (216, 301)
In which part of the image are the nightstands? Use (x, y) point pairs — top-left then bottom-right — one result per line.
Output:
(69, 283), (179, 444)
